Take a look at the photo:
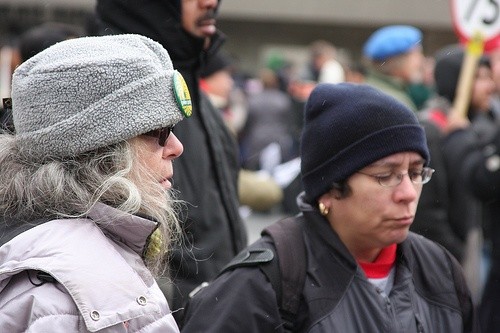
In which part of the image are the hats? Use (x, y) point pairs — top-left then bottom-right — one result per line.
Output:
(301, 82), (430, 203)
(11, 34), (192, 163)
(434, 45), (491, 102)
(363, 25), (422, 61)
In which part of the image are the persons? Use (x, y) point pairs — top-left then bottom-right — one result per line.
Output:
(197, 25), (500, 333)
(95, 0), (249, 333)
(0, 33), (193, 333)
(0, 24), (91, 135)
(176, 83), (474, 333)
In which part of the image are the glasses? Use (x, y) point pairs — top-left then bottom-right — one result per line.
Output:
(142, 124), (176, 146)
(352, 168), (435, 187)
